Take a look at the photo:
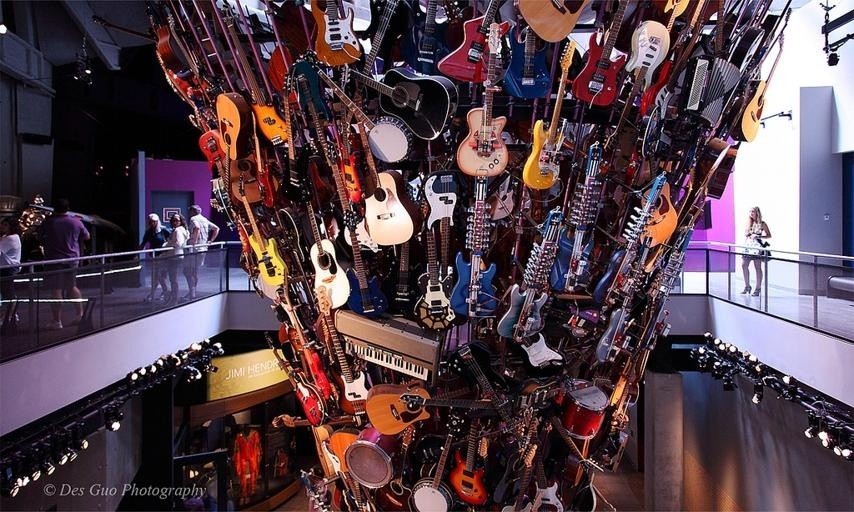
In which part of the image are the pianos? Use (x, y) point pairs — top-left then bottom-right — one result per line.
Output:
(334, 308), (438, 382)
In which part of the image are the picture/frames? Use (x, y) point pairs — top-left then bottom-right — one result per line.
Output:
(160, 206), (181, 224)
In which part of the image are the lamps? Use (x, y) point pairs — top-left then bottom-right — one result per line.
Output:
(686, 328), (854, 469)
(822, 46), (845, 68)
(0, 334), (225, 499)
(66, 29), (99, 85)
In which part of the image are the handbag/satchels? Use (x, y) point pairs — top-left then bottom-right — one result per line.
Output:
(761, 241), (772, 262)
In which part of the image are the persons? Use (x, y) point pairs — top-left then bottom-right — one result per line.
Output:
(0, 216), (25, 333)
(741, 206), (771, 299)
(208, 415), (235, 449)
(37, 198), (92, 330)
(232, 422), (263, 500)
(157, 213), (191, 305)
(183, 205), (220, 299)
(137, 212), (172, 302)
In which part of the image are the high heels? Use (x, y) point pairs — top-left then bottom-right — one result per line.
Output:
(752, 288), (761, 296)
(740, 286), (751, 294)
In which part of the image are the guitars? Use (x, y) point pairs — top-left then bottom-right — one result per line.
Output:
(146, 2), (781, 502)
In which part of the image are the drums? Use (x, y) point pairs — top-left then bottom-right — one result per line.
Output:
(345, 424), (399, 492)
(565, 376), (609, 442)
